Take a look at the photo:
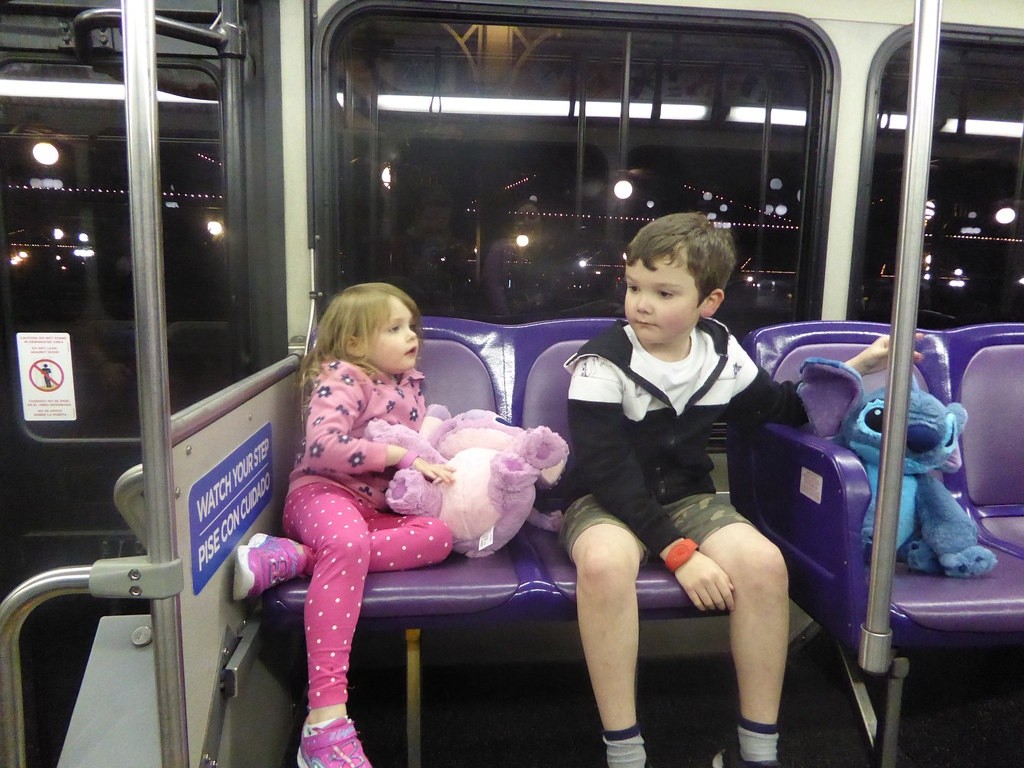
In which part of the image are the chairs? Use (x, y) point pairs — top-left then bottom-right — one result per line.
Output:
(268, 314), (1024, 768)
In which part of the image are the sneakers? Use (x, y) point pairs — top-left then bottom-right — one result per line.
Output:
(233, 532), (308, 602)
(296, 714), (373, 768)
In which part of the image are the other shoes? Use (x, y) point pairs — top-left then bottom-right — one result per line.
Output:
(712, 742), (781, 768)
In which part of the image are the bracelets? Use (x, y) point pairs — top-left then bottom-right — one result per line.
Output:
(664, 537), (697, 572)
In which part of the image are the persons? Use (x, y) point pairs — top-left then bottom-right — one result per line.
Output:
(233, 280), (454, 767)
(387, 194), (579, 326)
(563, 211), (926, 768)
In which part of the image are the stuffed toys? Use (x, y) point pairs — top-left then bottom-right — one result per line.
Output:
(796, 357), (999, 579)
(366, 402), (570, 560)
